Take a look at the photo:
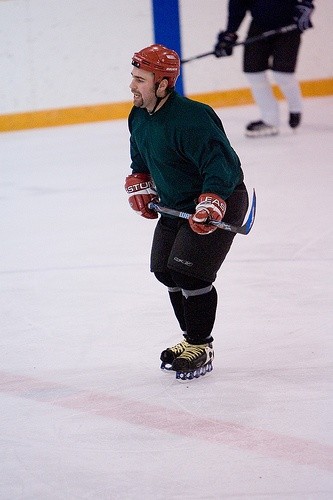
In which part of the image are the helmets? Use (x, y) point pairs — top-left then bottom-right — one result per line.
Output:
(130, 44), (181, 89)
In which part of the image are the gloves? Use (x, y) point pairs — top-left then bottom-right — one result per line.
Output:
(125, 173), (161, 219)
(187, 194), (227, 234)
(293, 2), (314, 32)
(214, 31), (237, 57)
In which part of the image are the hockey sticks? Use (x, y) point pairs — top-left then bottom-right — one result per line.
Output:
(150, 187), (258, 235)
(180, 26), (298, 64)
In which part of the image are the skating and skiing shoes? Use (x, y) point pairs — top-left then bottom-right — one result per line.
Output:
(172, 344), (215, 381)
(160, 338), (189, 372)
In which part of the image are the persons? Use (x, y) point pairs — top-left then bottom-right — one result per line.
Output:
(214, 0), (317, 137)
(122, 43), (247, 381)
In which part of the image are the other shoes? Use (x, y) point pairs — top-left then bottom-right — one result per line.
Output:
(246, 121), (280, 136)
(288, 112), (303, 129)
